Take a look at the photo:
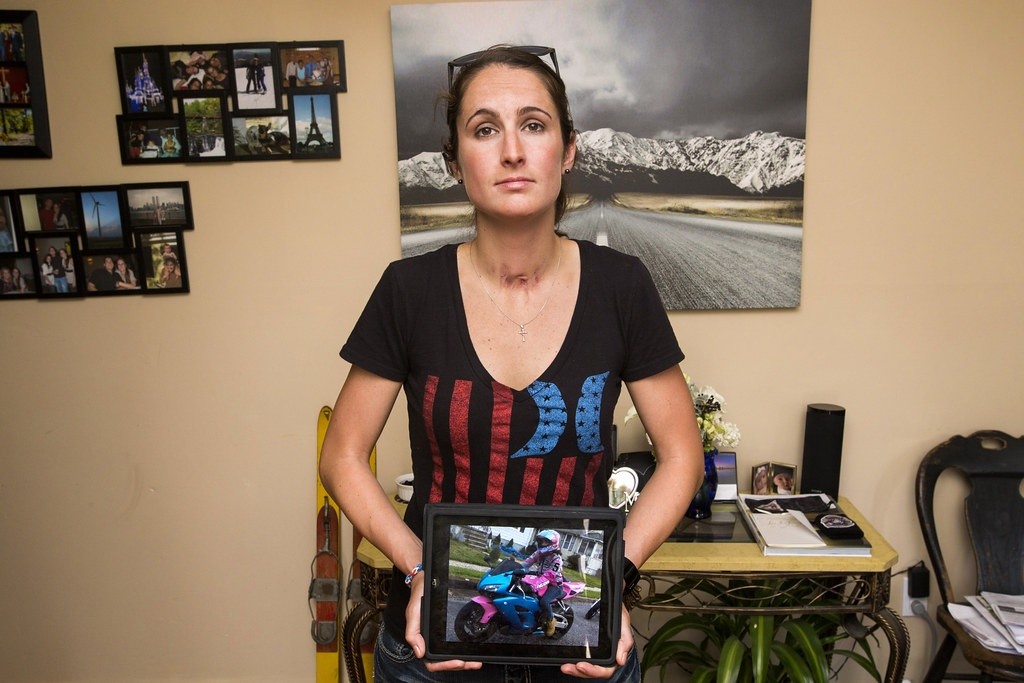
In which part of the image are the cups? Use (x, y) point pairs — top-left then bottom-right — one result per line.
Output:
(54, 269), (59, 274)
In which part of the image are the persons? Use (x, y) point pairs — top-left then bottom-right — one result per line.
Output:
(0, 24), (30, 103)
(0, 196), (182, 294)
(509, 529), (565, 636)
(128, 48), (334, 157)
(752, 466), (792, 497)
(317, 47), (704, 683)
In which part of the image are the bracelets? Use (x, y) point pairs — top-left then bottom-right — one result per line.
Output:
(404, 561), (422, 589)
(620, 553), (640, 595)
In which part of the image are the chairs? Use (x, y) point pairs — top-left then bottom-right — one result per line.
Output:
(915, 430), (1024, 683)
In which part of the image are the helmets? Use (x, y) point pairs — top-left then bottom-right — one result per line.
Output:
(536, 530), (560, 553)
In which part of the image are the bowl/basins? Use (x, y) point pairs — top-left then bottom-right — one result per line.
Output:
(394, 473), (414, 502)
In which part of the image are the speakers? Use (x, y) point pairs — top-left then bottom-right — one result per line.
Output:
(800, 404), (845, 502)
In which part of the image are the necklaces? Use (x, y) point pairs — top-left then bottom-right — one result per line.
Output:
(470, 238), (563, 342)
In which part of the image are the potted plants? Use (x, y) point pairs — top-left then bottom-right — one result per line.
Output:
(626, 570), (888, 683)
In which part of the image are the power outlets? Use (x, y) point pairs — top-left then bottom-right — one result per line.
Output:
(902, 576), (929, 616)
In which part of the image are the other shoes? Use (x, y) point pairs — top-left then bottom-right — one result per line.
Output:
(547, 618), (556, 636)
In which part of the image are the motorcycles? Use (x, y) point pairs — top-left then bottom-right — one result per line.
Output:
(586, 598), (602, 620)
(455, 546), (574, 643)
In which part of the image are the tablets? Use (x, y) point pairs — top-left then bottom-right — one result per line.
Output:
(423, 504), (626, 665)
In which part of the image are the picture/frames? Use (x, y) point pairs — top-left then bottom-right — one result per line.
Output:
(0, 181), (195, 298)
(0, 9), (53, 159)
(419, 505), (624, 669)
(115, 40), (347, 166)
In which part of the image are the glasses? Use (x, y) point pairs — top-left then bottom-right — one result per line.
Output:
(447, 45), (559, 93)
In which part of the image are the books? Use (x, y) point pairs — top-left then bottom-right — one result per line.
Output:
(732, 491), (872, 558)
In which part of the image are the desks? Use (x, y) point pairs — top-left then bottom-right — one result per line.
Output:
(339, 490), (910, 683)
